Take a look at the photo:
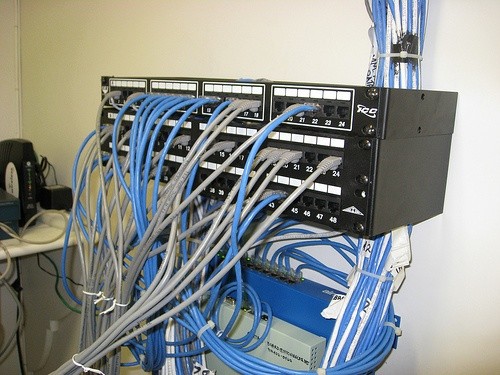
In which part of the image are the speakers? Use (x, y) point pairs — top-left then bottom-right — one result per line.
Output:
(42, 184), (73, 211)
(0, 188), (22, 241)
